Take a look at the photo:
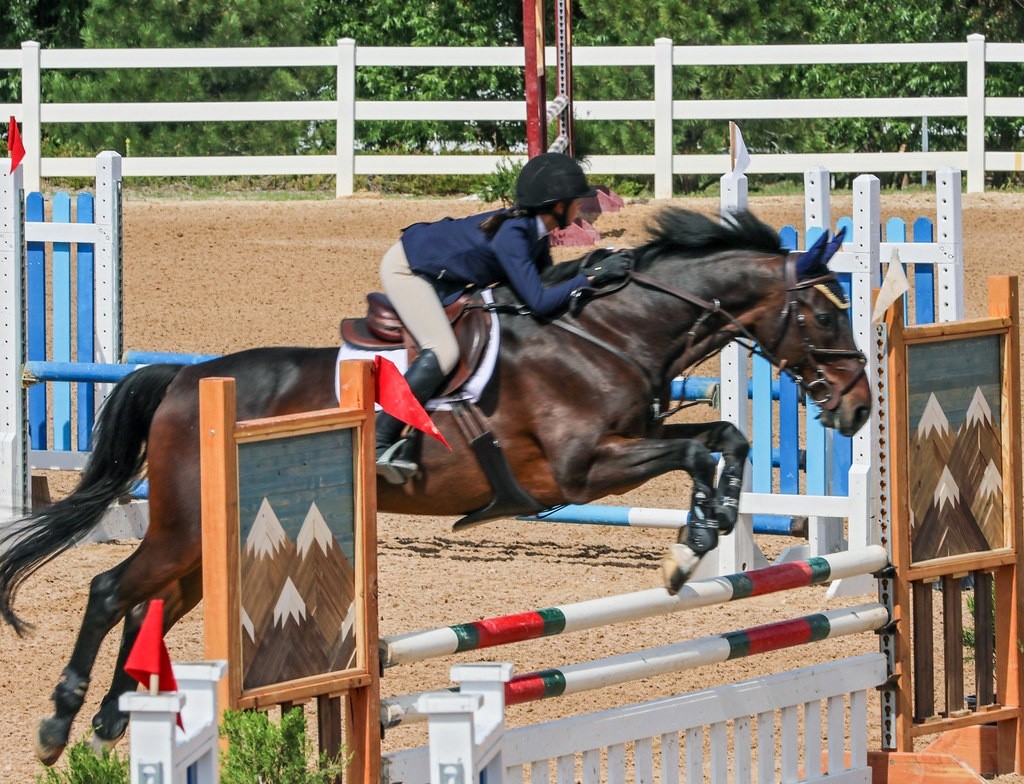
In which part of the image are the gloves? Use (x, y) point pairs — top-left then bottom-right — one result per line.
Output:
(583, 251), (633, 285)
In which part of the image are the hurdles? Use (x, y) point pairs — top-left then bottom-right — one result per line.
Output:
(185, 267), (1024, 784)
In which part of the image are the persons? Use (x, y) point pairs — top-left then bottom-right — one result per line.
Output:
(366, 152), (646, 482)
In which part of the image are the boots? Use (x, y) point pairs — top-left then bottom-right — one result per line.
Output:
(376, 349), (446, 486)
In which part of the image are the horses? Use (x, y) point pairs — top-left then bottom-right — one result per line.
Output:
(1, 202), (874, 766)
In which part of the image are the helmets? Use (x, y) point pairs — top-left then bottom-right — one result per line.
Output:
(517, 152), (599, 206)
